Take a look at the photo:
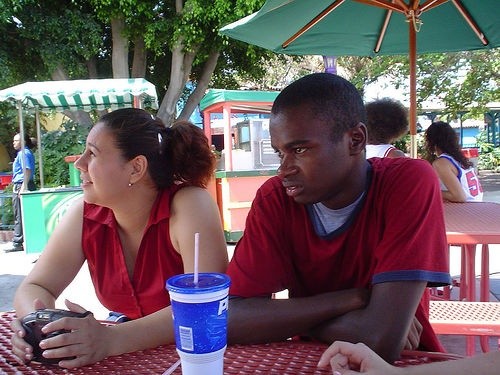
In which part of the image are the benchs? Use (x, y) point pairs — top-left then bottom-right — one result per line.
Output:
(427, 301), (500, 355)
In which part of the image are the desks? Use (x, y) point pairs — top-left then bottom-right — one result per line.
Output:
(442, 199), (500, 302)
(0, 311), (466, 375)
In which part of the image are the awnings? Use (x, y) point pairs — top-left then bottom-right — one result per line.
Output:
(449, 118), (486, 129)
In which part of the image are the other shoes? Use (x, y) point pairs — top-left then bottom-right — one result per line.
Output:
(3, 246), (24, 251)
(430, 285), (452, 294)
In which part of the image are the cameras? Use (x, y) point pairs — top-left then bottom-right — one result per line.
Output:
(20, 309), (86, 364)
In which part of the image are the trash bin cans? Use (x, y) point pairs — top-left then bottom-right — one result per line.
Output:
(460, 147), (480, 177)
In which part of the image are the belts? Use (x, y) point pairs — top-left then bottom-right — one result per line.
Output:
(14, 182), (22, 184)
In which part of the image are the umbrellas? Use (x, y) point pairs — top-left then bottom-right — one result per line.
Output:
(219, 0), (500, 159)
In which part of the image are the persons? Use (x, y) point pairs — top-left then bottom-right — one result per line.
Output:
(3, 133), (34, 252)
(317, 342), (500, 375)
(9, 108), (228, 369)
(425, 121), (483, 203)
(225, 73), (450, 363)
(362, 98), (409, 160)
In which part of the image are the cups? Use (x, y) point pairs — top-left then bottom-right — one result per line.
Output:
(165, 273), (231, 375)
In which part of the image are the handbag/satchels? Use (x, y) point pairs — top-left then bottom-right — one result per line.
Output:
(28, 180), (36, 190)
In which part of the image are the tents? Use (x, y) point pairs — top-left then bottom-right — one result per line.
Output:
(0, 78), (159, 192)
